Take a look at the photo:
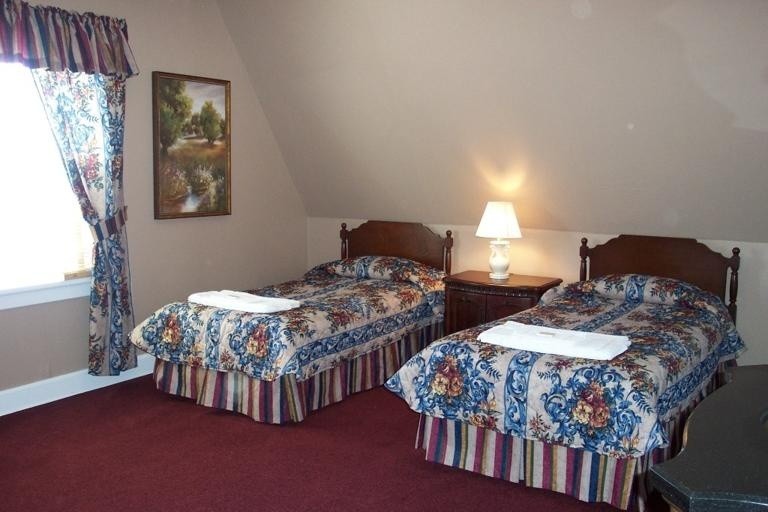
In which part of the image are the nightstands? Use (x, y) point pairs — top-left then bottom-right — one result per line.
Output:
(443, 270), (562, 336)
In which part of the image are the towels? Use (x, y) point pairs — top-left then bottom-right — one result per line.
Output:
(477, 320), (631, 362)
(187, 290), (302, 314)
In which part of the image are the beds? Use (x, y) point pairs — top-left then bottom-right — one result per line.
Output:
(384, 234), (740, 511)
(126, 219), (454, 425)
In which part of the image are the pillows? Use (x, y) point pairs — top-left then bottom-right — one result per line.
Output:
(325, 254), (447, 286)
(564, 270), (747, 358)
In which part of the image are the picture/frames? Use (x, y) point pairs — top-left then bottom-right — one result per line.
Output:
(152, 71), (231, 219)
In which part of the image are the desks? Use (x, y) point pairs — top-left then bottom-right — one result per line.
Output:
(647, 365), (768, 512)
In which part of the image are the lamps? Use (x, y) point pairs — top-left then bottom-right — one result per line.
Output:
(475, 201), (522, 280)
